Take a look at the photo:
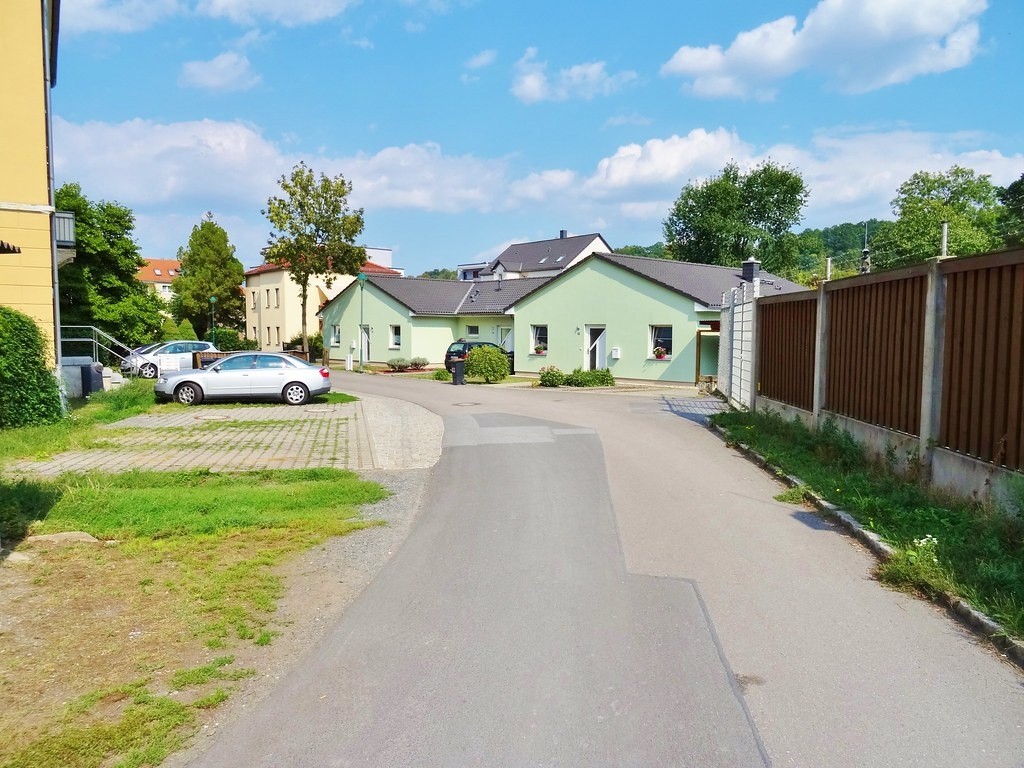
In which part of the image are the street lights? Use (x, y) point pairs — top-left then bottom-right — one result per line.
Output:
(356, 273), (367, 373)
(210, 297), (216, 346)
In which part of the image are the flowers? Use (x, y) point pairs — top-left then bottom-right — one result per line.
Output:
(653, 347), (666, 355)
(541, 342), (547, 350)
(535, 344), (543, 351)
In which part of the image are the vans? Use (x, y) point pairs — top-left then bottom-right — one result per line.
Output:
(445, 342), (514, 375)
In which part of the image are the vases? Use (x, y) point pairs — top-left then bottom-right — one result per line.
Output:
(656, 354), (665, 359)
(536, 350), (542, 354)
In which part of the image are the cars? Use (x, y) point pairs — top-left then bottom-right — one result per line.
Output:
(154, 352), (331, 406)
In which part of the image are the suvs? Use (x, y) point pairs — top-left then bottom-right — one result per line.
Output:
(121, 341), (220, 379)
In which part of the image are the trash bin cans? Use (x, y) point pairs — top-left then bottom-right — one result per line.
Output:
(450, 358), (467, 385)
